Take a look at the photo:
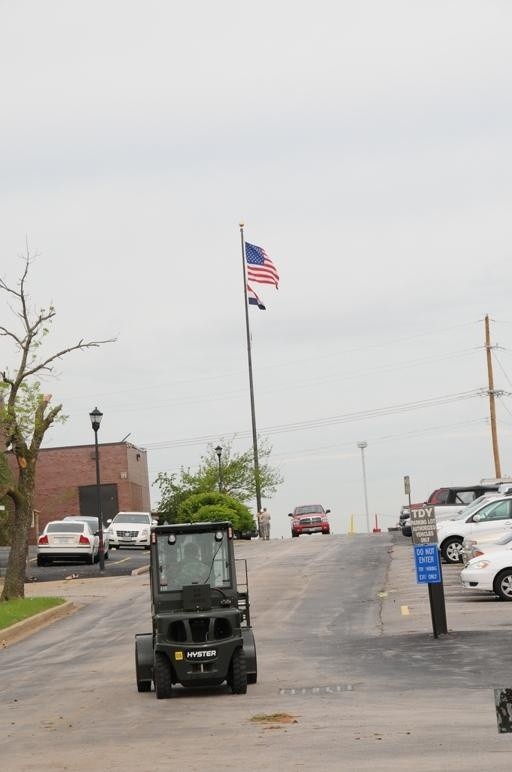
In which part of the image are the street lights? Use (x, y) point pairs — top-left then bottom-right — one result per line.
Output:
(214, 445), (225, 495)
(88, 406), (105, 574)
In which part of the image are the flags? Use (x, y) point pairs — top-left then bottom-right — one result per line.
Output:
(243, 240), (279, 292)
(247, 286), (266, 311)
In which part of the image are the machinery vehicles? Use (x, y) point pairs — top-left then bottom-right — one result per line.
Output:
(132, 520), (257, 699)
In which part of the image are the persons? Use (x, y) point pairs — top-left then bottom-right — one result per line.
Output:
(255, 508), (265, 538)
(261, 507), (272, 541)
(168, 541), (216, 590)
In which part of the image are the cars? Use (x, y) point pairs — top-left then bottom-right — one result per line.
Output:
(288, 504), (331, 537)
(398, 477), (512, 602)
(35, 511), (158, 566)
